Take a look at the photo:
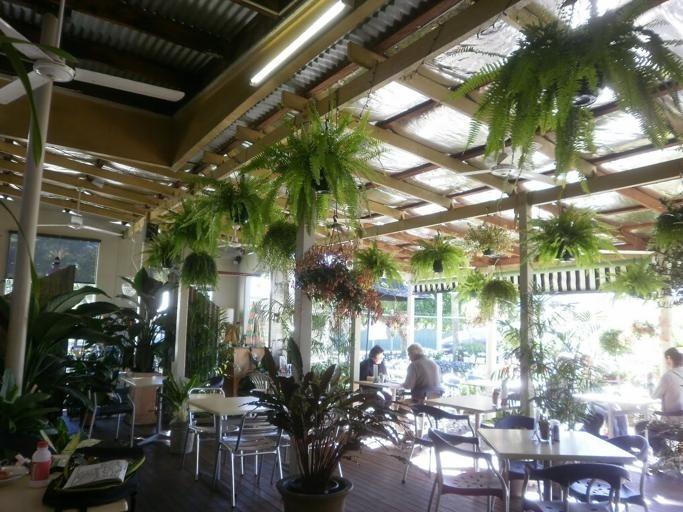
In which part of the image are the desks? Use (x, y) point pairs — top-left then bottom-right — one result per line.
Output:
(423, 396), (521, 473)
(118, 375), (191, 447)
(188, 395), (278, 499)
(355, 381), (405, 441)
(476, 429), (636, 512)
(571, 393), (663, 438)
(218, 346), (271, 397)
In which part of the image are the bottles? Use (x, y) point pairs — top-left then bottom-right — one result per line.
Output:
(31, 442), (49, 487)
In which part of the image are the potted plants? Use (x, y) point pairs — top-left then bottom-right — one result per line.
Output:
(462, 223), (514, 259)
(235, 335), (417, 512)
(148, 368), (211, 454)
(176, 170), (284, 259)
(139, 225), (184, 271)
(239, 90), (387, 238)
(180, 250), (219, 292)
(158, 197), (217, 262)
(479, 280), (518, 321)
(252, 217), (297, 276)
(648, 198), (683, 261)
(447, 0), (679, 194)
(515, 201), (625, 272)
(110, 308), (141, 371)
(0, 199), (123, 467)
(352, 240), (402, 285)
(108, 265), (172, 373)
(599, 255), (668, 300)
(458, 271), (487, 300)
(411, 236), (470, 286)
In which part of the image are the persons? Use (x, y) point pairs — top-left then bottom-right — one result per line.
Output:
(645, 347), (683, 459)
(361, 345), (393, 409)
(393, 342), (440, 434)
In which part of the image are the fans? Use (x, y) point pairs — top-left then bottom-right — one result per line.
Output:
(216, 223), (257, 249)
(0, 23), (184, 106)
(455, 141), (560, 186)
(36, 186), (123, 237)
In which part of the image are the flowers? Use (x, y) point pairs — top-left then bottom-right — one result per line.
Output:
(294, 243), (385, 322)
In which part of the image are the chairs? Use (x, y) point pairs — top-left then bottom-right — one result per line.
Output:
(643, 411), (683, 478)
(81, 370), (136, 450)
(519, 463), (631, 512)
(270, 399), (344, 485)
(181, 388), (239, 481)
(492, 416), (544, 512)
(393, 386), (445, 451)
(249, 373), (275, 397)
(401, 404), (475, 484)
(568, 435), (648, 511)
(249, 389), (292, 477)
(213, 402), (289, 509)
(428, 427), (509, 512)
